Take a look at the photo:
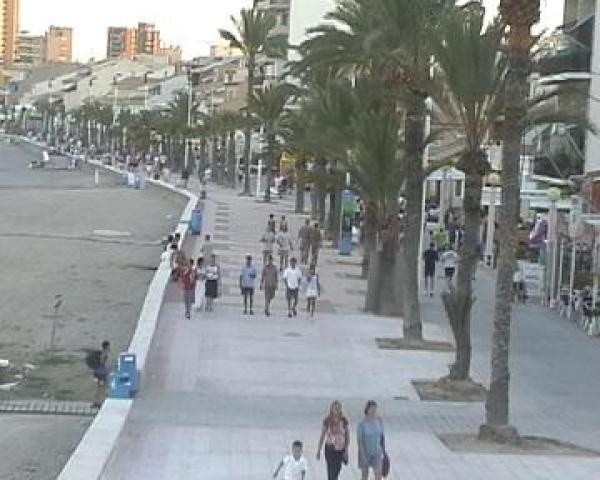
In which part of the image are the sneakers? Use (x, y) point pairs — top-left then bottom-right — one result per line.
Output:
(244, 308), (298, 317)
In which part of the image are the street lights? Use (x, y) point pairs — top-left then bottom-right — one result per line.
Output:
(144, 71), (154, 110)
(89, 76), (98, 106)
(112, 72), (124, 105)
(484, 173), (500, 271)
(546, 187), (561, 308)
(49, 77), (57, 106)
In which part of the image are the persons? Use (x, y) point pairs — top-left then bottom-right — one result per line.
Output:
(317, 400), (350, 480)
(273, 439), (308, 480)
(26, 131), (207, 199)
(356, 398), (387, 480)
(161, 231), (221, 320)
(275, 174), (285, 199)
(239, 211), (324, 320)
(89, 341), (112, 408)
(355, 194), (466, 298)
(512, 267), (523, 305)
(238, 168), (244, 183)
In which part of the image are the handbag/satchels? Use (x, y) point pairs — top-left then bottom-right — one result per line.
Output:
(382, 455), (389, 475)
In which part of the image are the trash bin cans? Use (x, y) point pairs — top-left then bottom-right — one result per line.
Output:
(338, 239), (352, 255)
(191, 210), (201, 235)
(108, 354), (137, 398)
